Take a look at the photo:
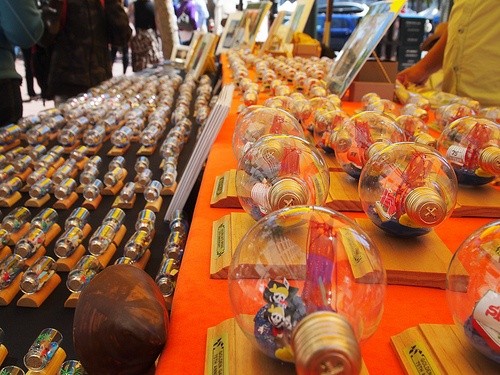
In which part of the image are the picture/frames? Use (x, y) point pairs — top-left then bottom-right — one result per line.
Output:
(170, 0), (315, 82)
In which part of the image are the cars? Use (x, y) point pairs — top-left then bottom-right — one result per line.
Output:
(312, 2), (369, 52)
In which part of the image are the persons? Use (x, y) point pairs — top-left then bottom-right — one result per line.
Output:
(395, 0), (500, 109)
(22, 47), (45, 100)
(110, 0), (130, 74)
(0, 0), (44, 128)
(129, 0), (160, 72)
(418, 20), (448, 90)
(175, 0), (199, 46)
(190, 0), (226, 33)
(44, 0), (113, 103)
